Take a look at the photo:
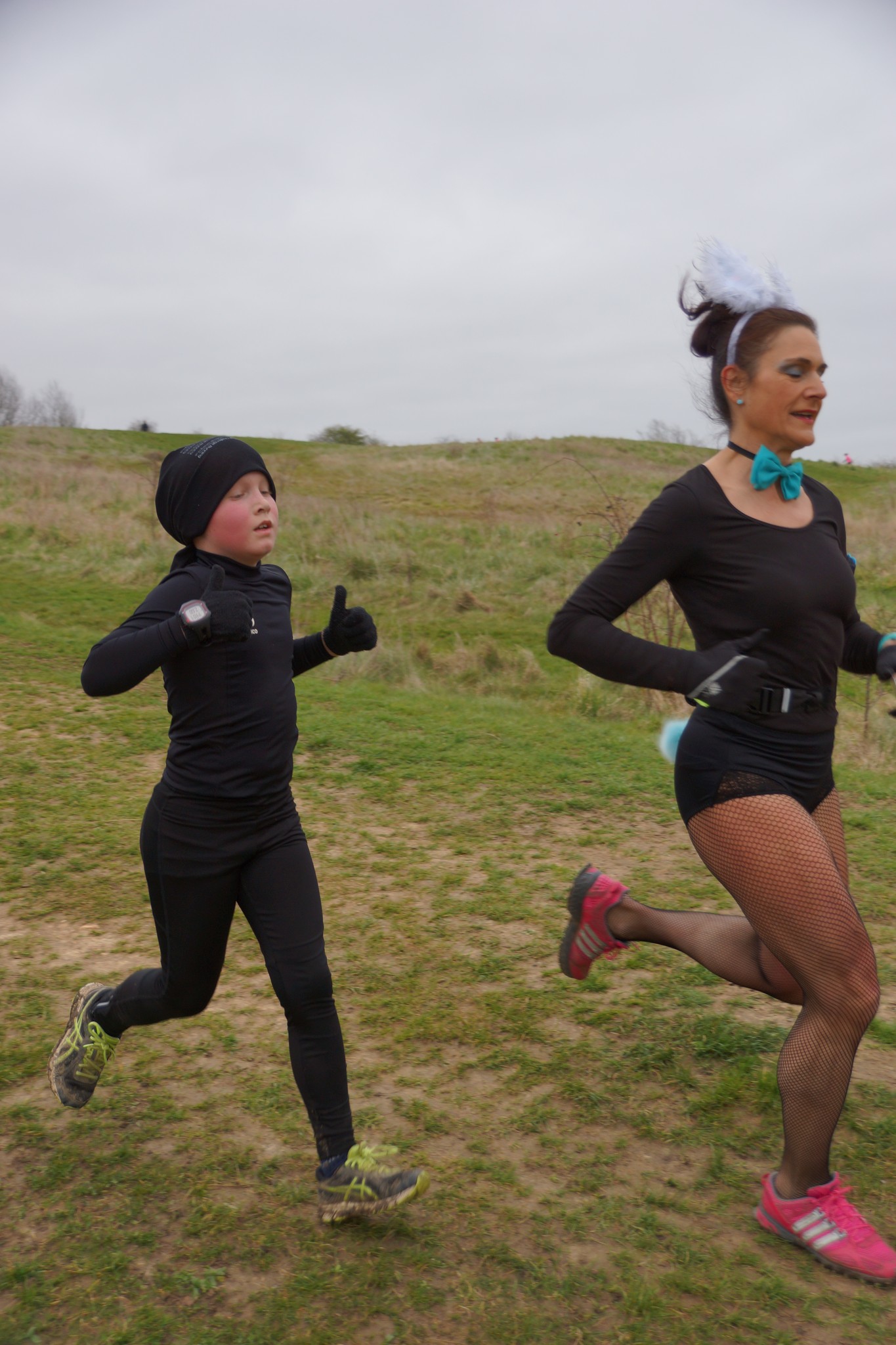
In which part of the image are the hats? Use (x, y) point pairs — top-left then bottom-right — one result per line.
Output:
(154, 435), (277, 545)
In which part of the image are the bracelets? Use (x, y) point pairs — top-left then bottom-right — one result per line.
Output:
(877, 632), (896, 654)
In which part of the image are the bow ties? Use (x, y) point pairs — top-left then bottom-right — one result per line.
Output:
(751, 446), (805, 500)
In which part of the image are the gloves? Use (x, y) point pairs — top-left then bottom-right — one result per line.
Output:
(879, 645), (896, 718)
(181, 564), (254, 648)
(685, 627), (771, 712)
(323, 582), (376, 654)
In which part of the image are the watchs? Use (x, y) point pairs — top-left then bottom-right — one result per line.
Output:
(179, 599), (214, 648)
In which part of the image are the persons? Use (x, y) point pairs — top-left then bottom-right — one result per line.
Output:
(559, 233), (896, 1286)
(46, 436), (430, 1224)
(843, 454), (852, 464)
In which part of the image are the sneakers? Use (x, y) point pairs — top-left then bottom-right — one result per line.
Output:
(756, 1170), (895, 1285)
(46, 983), (123, 1111)
(558, 863), (640, 981)
(316, 1140), (431, 1221)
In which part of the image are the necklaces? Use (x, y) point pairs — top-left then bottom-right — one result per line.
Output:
(725, 439), (805, 501)
